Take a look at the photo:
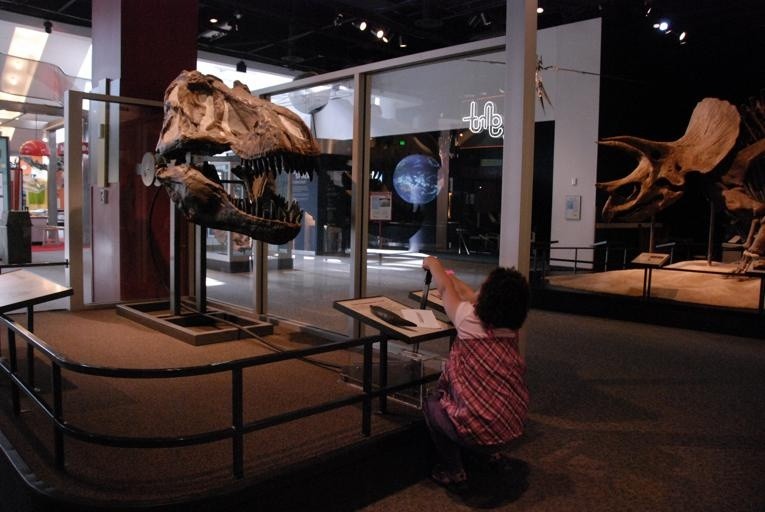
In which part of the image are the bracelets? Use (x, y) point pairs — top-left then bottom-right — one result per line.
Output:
(445, 269), (454, 274)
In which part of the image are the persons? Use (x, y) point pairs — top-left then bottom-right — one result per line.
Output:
(423, 257), (530, 484)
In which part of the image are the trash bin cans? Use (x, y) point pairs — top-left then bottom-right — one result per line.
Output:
(0, 210), (34, 265)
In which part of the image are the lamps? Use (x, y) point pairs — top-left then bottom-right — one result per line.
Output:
(641, 0), (689, 46)
(208, 10), (245, 32)
(331, 8), (409, 49)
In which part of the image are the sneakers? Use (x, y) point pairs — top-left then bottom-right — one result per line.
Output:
(432, 452), (512, 484)
